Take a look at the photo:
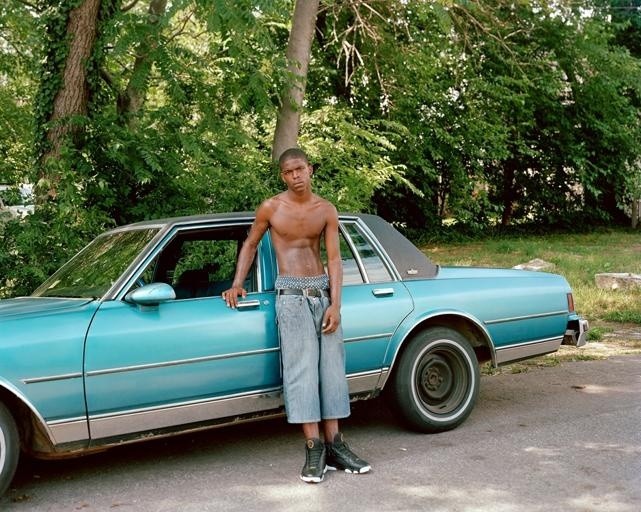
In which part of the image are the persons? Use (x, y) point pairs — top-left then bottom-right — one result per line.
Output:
(221, 148), (372, 483)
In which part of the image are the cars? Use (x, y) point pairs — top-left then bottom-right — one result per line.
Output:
(1, 211), (590, 506)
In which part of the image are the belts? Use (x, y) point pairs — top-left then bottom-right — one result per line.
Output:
(276, 288), (330, 297)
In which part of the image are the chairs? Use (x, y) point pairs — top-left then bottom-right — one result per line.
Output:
(169, 268), (210, 298)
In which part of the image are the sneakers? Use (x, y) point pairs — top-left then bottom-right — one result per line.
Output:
(325, 432), (371, 474)
(300, 438), (327, 482)
